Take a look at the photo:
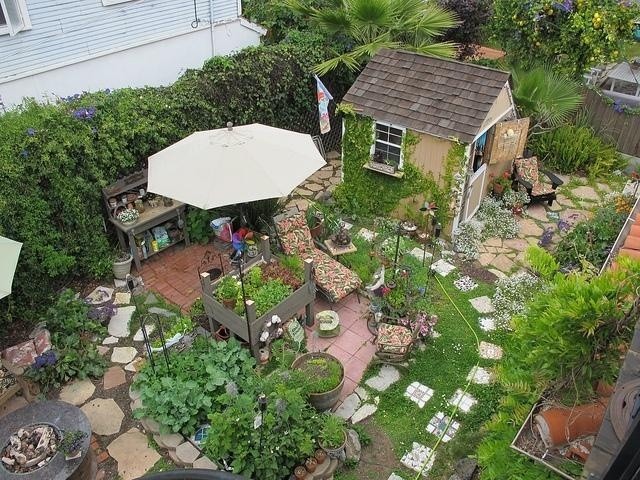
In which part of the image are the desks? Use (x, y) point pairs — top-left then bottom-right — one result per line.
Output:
(0, 401), (92, 480)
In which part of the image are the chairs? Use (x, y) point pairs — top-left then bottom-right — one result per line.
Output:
(271, 205), (362, 310)
(366, 312), (432, 363)
(511, 155), (564, 209)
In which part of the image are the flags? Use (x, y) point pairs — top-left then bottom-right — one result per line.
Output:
(317, 82), (330, 135)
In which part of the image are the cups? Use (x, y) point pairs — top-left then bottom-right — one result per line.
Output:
(139, 188), (145, 195)
(152, 241), (160, 253)
(122, 198), (126, 202)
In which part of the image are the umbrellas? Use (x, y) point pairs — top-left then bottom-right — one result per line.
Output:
(146, 122), (328, 223)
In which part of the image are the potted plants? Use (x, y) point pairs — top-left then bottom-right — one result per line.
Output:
(399, 203), (421, 238)
(291, 352), (344, 410)
(112, 242), (133, 279)
(318, 416), (347, 453)
(487, 178), (510, 194)
(416, 213), (430, 243)
(53, 429), (88, 457)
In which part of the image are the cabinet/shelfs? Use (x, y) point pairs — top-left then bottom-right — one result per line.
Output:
(102, 170), (191, 272)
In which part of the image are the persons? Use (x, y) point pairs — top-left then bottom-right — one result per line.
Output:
(231, 228), (245, 261)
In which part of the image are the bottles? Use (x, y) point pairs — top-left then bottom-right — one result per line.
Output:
(142, 244), (149, 260)
(109, 197), (119, 207)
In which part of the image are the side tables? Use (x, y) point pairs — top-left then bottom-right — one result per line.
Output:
(324, 238), (357, 267)
